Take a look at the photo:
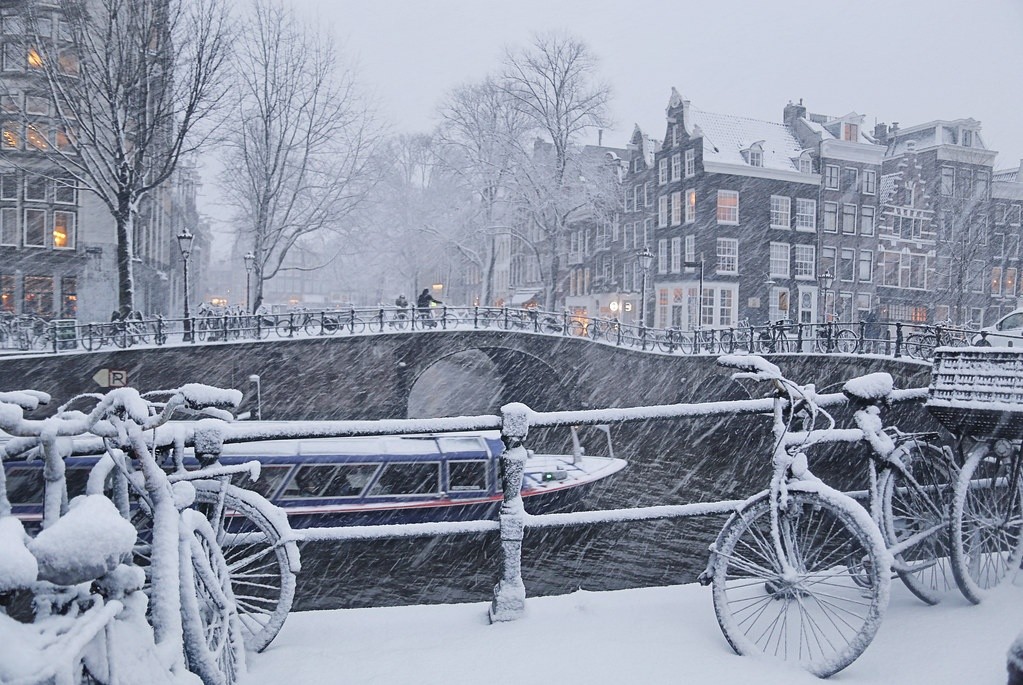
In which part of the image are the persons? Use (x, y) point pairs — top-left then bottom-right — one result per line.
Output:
(389, 295), (408, 328)
(417, 289), (442, 330)
(380, 464), (486, 493)
(295, 466), (352, 496)
(245, 471), (270, 499)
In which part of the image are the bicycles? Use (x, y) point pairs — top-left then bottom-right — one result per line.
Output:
(719, 316), (756, 353)
(197, 303), (272, 342)
(321, 304), (365, 335)
(539, 309), (585, 337)
(658, 327), (693, 355)
(925, 305), (1023, 605)
(585, 316), (626, 343)
(368, 303), (411, 333)
(621, 319), (655, 352)
(815, 312), (859, 352)
(905, 318), (974, 363)
(0, 377), (300, 685)
(690, 324), (721, 354)
(274, 306), (321, 337)
(757, 318), (793, 352)
(413, 302), (542, 332)
(694, 355), (984, 680)
(0, 310), (169, 352)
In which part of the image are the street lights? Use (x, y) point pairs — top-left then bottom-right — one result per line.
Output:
(637, 248), (655, 339)
(818, 269), (835, 325)
(244, 252), (255, 318)
(175, 227), (194, 342)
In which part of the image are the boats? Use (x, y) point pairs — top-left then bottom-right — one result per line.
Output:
(2, 411), (627, 569)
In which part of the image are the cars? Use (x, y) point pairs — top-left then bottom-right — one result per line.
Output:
(971, 308), (1023, 349)
